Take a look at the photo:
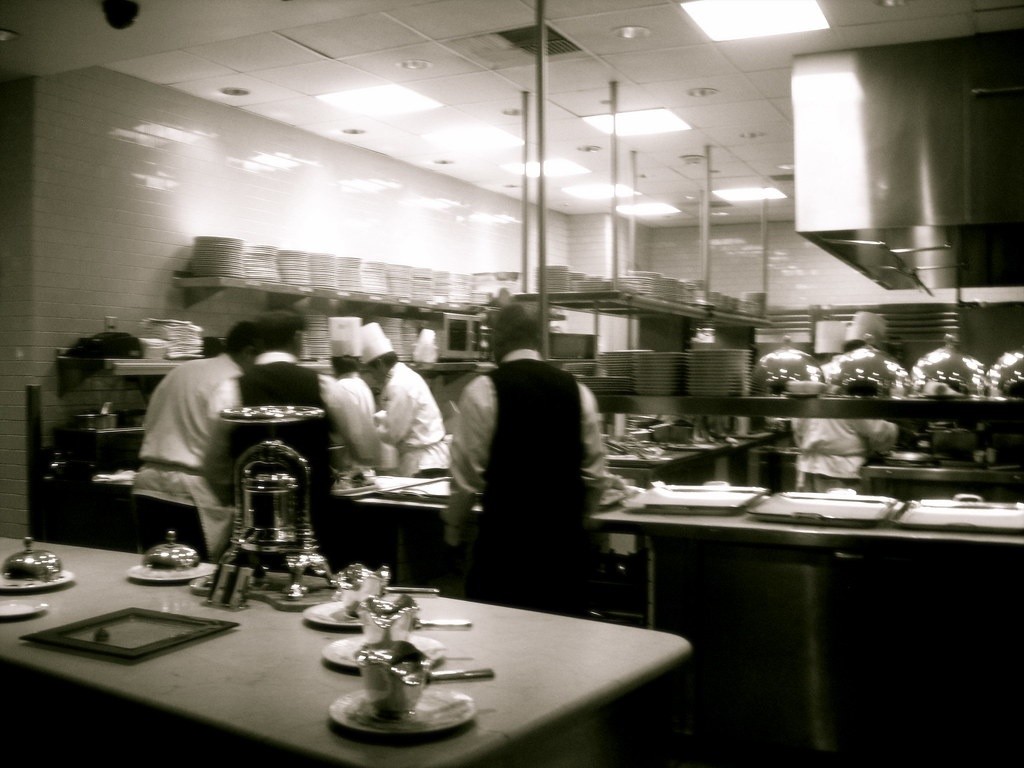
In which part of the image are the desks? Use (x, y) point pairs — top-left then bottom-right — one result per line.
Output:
(0, 536), (693, 768)
(859, 464), (1024, 502)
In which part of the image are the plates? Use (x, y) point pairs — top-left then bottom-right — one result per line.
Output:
(0, 600), (49, 618)
(302, 600), (365, 626)
(126, 562), (217, 582)
(374, 315), (429, 362)
(323, 633), (445, 670)
(329, 688), (476, 736)
(190, 234), (494, 306)
(533, 264), (768, 317)
(294, 314), (333, 364)
(0, 570), (75, 590)
(560, 348), (754, 397)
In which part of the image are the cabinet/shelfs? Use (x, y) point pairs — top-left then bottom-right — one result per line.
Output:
(170, 275), (487, 373)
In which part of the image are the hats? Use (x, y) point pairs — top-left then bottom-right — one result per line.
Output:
(357, 322), (394, 366)
(844, 311), (887, 350)
(326, 316), (363, 358)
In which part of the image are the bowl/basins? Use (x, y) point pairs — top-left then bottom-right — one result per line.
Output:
(549, 332), (599, 359)
(494, 272), (519, 281)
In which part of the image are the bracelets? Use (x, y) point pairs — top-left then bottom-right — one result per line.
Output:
(443, 523), (467, 529)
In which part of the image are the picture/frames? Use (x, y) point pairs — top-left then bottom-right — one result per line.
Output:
(19, 606), (242, 663)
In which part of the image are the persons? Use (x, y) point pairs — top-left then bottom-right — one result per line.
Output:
(790, 310), (898, 495)
(131, 309), (383, 576)
(330, 317), (451, 588)
(442, 302), (607, 620)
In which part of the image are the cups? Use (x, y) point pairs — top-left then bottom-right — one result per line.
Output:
(972, 448), (997, 464)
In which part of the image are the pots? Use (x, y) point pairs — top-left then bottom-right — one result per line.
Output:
(71, 408), (117, 428)
